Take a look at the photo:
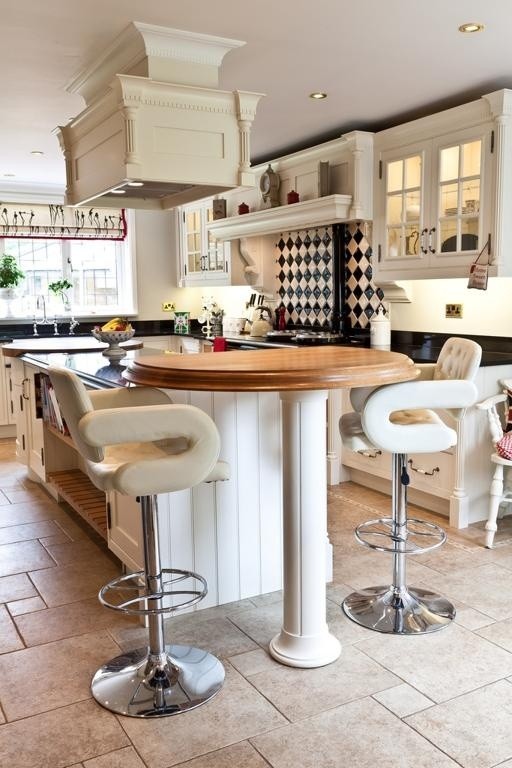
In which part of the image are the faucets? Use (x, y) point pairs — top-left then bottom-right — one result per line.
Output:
(35, 294), (54, 325)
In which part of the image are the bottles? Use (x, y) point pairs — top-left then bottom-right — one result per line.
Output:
(287, 190), (299, 204)
(238, 202), (249, 215)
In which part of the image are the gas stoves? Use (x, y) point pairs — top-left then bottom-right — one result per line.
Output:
(263, 328), (365, 347)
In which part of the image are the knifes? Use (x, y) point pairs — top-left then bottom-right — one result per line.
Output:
(250, 292), (264, 306)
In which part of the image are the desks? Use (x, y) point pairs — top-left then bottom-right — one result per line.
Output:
(122, 348), (422, 666)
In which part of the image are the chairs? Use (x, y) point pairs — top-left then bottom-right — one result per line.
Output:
(338, 335), (485, 634)
(45, 365), (227, 717)
(476, 377), (512, 549)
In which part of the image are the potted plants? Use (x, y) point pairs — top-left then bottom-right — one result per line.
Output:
(0, 252), (25, 301)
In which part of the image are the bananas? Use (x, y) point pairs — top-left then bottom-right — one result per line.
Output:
(101, 316), (122, 332)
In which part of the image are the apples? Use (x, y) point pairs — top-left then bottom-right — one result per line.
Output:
(117, 323), (126, 331)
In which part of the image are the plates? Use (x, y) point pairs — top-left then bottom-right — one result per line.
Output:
(444, 206), (471, 215)
(441, 233), (479, 252)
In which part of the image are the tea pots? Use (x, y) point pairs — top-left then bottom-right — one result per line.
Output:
(405, 231), (419, 255)
(245, 305), (275, 336)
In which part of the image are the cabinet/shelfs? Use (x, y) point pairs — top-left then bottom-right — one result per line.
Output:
(9, 357), (331, 627)
(176, 195), (250, 288)
(332, 387), (512, 530)
(371, 101), (512, 286)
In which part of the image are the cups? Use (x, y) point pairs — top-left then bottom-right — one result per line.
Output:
(465, 199), (480, 211)
(234, 317), (246, 334)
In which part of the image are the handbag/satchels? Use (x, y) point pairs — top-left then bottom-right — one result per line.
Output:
(467, 241), (490, 290)
